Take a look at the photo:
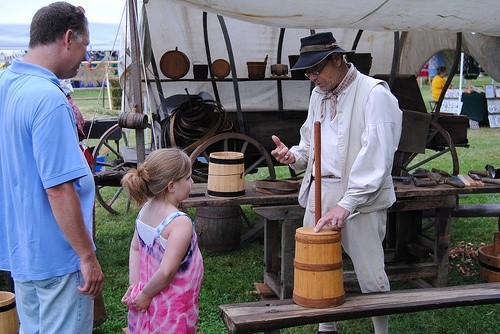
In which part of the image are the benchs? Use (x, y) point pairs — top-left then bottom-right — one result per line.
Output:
(421, 204), (500, 237)
(218, 281), (500, 334)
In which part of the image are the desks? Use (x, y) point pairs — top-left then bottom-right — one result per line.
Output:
(181, 174), (500, 301)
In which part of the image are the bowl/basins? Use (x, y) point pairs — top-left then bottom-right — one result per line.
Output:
(193, 64), (208, 79)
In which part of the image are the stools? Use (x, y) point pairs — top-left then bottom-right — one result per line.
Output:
(429, 100), (437, 112)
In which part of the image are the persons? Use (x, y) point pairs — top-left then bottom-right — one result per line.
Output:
(271, 31), (403, 334)
(431, 66), (452, 101)
(121, 148), (204, 334)
(0, 0), (105, 334)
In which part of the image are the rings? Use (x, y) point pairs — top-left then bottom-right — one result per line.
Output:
(332, 220), (337, 225)
(285, 156), (289, 159)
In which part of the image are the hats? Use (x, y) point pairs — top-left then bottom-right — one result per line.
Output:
(289, 31), (356, 71)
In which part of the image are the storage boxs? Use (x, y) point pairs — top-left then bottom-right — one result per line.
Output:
(430, 114), (470, 146)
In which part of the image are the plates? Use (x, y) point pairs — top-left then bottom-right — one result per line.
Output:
(212, 59), (231, 78)
(159, 50), (190, 79)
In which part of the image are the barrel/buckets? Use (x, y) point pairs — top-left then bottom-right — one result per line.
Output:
(293, 226), (344, 308)
(288, 55), (305, 77)
(0, 291), (20, 334)
(478, 232), (500, 282)
(206, 153), (245, 198)
(247, 62), (267, 78)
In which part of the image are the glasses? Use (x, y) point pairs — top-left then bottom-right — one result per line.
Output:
(305, 59), (331, 78)
